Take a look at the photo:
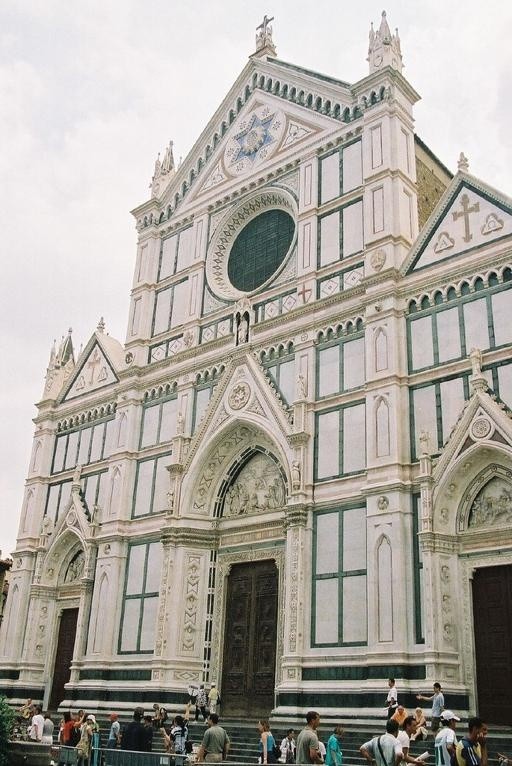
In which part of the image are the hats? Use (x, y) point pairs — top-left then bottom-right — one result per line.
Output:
(210, 682), (217, 687)
(440, 711), (461, 723)
(87, 714), (97, 724)
(109, 714), (120, 721)
(144, 716), (153, 723)
(199, 681), (205, 687)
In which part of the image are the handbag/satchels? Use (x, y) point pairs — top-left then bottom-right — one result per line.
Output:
(217, 698), (221, 705)
(384, 700), (397, 706)
(184, 740), (192, 760)
(272, 744), (281, 758)
(69, 727), (81, 747)
(222, 744), (227, 760)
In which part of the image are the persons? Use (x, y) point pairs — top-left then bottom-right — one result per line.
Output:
(416, 682), (445, 747)
(14, 676), (231, 765)
(383, 676), (400, 720)
(249, 705), (494, 766)
